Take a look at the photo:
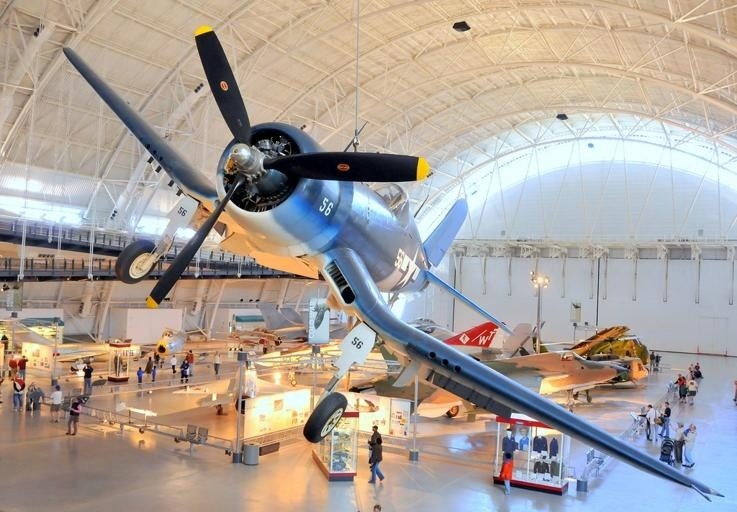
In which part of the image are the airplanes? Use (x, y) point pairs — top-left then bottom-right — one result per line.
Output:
(63, 24), (725, 502)
(11, 322), (282, 371)
(349, 318), (649, 419)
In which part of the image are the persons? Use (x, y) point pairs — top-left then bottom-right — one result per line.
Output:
(645, 351), (704, 468)
(503, 427), (561, 481)
(500, 453), (513, 495)
(367, 425), (385, 484)
(211, 350), (222, 375)
(0, 333), (194, 436)
(373, 505), (382, 512)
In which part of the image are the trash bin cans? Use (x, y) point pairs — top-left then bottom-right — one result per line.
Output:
(243, 441), (259, 466)
